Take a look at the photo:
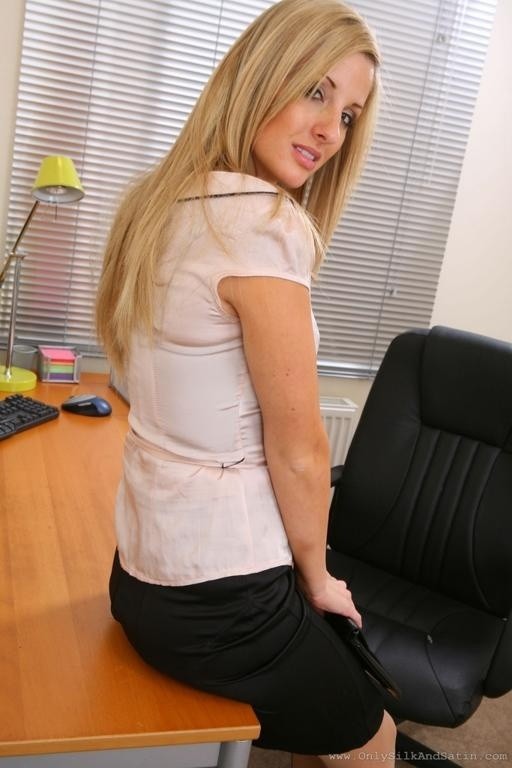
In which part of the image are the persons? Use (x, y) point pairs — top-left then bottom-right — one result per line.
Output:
(92, 1), (399, 767)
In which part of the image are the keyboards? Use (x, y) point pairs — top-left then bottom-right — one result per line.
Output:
(0, 392), (60, 441)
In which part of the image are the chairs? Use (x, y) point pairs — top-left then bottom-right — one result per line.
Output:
(324, 325), (512, 768)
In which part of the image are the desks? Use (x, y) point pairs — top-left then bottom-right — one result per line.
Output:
(0, 370), (262, 768)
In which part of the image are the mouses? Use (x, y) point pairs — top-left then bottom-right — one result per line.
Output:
(61, 392), (112, 416)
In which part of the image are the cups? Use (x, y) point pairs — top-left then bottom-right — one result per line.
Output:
(12, 344), (37, 371)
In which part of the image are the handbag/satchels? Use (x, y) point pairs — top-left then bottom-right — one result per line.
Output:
(326, 611), (403, 703)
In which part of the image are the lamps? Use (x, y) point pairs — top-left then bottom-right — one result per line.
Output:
(1, 153), (86, 395)
